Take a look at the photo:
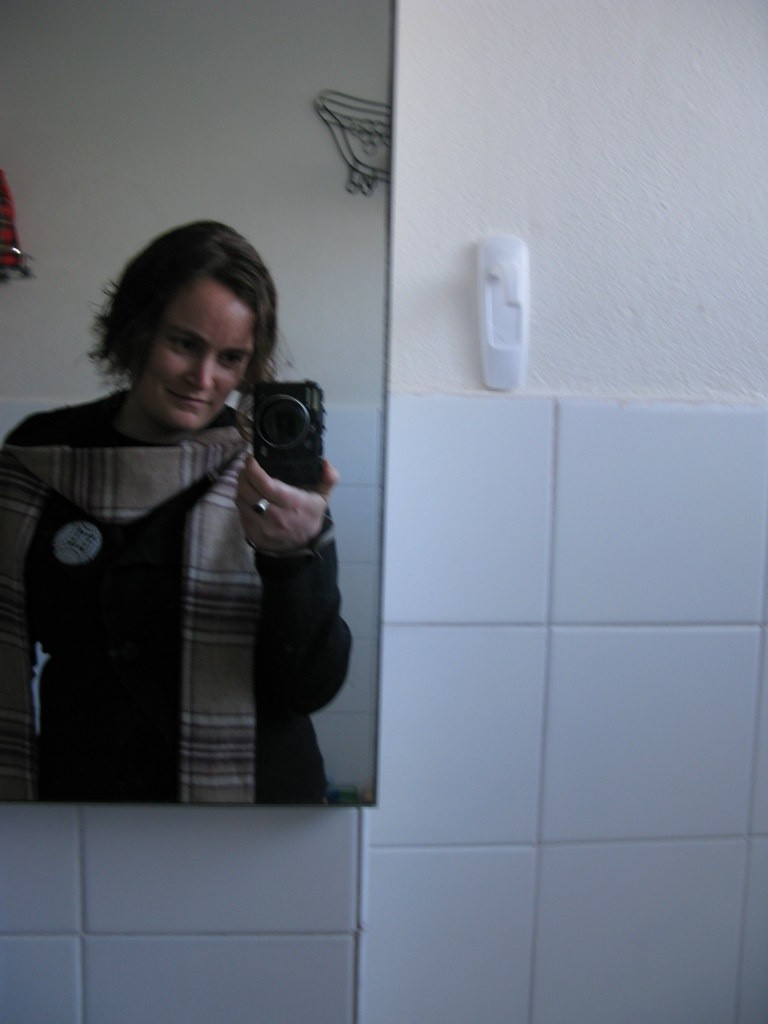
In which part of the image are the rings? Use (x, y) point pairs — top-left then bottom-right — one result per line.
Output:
(254, 498), (269, 515)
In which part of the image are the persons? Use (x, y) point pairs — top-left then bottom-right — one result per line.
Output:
(0, 220), (353, 801)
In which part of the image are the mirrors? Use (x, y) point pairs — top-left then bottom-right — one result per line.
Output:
(0, 0), (398, 810)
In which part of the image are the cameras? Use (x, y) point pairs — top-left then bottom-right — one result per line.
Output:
(254, 380), (326, 485)
